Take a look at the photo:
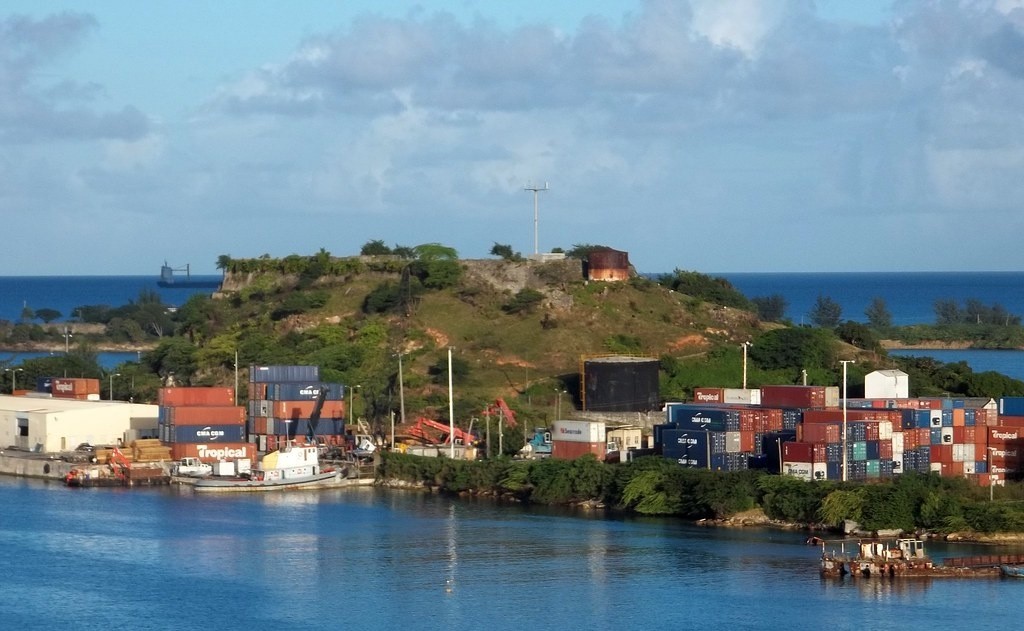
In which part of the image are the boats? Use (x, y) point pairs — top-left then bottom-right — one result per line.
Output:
(391, 411), (478, 461)
(168, 420), (348, 492)
(848, 538), (933, 578)
(157, 261), (222, 288)
(1000, 565), (1024, 579)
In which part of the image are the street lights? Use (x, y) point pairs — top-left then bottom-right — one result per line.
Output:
(5, 368), (23, 393)
(344, 384), (360, 424)
(62, 334), (73, 354)
(109, 374), (121, 400)
(839, 360), (855, 481)
(446, 345), (455, 458)
(392, 353), (406, 423)
(987, 447), (997, 500)
(740, 341), (753, 389)
(554, 388), (567, 420)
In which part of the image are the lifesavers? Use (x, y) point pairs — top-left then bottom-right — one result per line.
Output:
(297, 469), (302, 474)
(274, 471), (279, 476)
